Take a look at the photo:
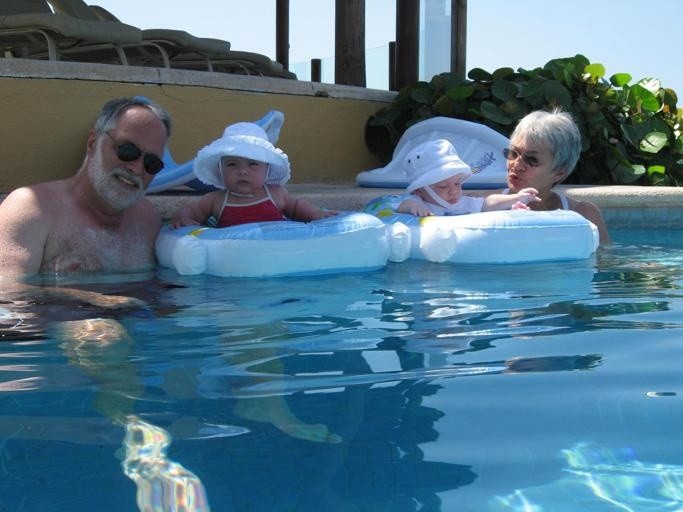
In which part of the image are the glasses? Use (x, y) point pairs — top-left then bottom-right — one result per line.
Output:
(502, 149), (553, 171)
(104, 129), (165, 174)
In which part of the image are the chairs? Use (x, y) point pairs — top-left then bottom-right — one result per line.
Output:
(0, 1), (298, 80)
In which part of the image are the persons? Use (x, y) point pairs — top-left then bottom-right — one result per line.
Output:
(171, 121), (340, 229)
(397, 137), (542, 217)
(484, 104), (611, 244)
(0, 95), (173, 312)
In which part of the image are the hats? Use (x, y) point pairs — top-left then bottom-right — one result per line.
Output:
(193, 122), (291, 190)
(402, 139), (474, 195)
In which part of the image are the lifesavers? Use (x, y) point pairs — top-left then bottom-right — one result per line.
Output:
(362, 196), (599, 264)
(155, 210), (388, 277)
